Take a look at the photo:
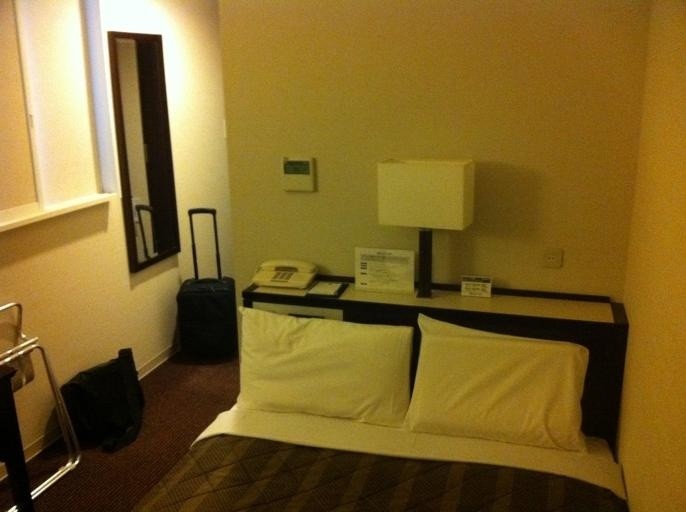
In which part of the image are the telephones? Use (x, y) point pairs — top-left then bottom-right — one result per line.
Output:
(251, 259), (320, 290)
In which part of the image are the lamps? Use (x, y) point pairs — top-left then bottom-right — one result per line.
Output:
(377, 161), (477, 298)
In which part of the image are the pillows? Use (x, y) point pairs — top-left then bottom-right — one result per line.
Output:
(403, 313), (590, 455)
(239, 305), (414, 428)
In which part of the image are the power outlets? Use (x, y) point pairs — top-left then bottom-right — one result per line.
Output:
(542, 247), (563, 268)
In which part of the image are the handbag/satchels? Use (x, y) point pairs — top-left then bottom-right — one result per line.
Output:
(60, 348), (144, 453)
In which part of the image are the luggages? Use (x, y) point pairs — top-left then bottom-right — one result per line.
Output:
(176, 208), (238, 365)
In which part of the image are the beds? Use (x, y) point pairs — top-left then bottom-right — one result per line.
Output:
(132, 274), (628, 512)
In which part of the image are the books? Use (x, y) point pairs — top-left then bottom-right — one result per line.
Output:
(305, 279), (350, 299)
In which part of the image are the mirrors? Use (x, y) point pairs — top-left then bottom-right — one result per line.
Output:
(109, 31), (181, 272)
